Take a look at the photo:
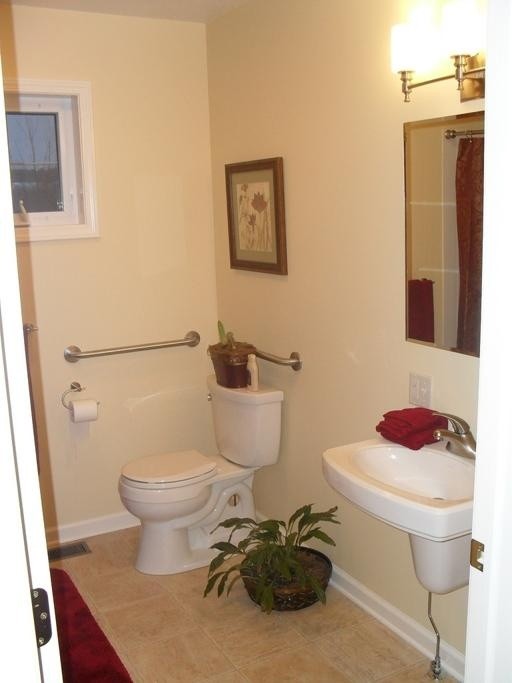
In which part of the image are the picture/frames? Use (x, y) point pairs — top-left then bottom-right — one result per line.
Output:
(3, 74), (97, 238)
(224, 155), (292, 274)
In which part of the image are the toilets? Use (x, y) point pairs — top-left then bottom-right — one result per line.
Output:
(117, 374), (284, 576)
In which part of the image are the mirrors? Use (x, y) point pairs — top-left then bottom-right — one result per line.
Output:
(403, 110), (484, 357)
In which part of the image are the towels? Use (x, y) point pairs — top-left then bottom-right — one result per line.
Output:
(407, 276), (435, 344)
(50, 568), (134, 683)
(376, 406), (448, 451)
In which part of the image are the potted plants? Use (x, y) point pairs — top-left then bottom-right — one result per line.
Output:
(203, 502), (340, 612)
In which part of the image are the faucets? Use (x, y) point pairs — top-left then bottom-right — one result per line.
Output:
(431, 411), (476, 461)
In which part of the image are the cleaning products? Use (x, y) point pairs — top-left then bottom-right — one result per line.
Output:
(245, 353), (259, 391)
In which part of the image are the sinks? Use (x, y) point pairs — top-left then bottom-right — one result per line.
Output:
(324, 436), (475, 541)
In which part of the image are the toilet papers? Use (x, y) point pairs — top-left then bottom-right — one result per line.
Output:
(68, 399), (99, 423)
